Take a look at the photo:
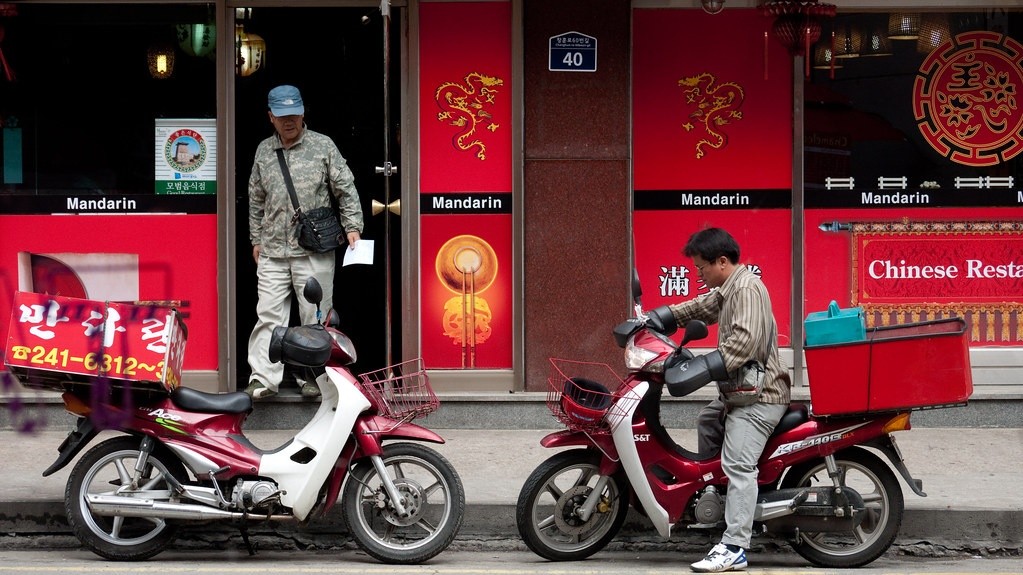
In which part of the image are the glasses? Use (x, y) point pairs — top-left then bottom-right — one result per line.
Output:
(695, 260), (712, 275)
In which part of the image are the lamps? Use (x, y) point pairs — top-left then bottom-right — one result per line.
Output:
(813, 16), (843, 70)
(832, 13), (867, 59)
(859, 14), (894, 57)
(917, 13), (958, 55)
(145, 48), (174, 79)
(230, 6), (267, 77)
(886, 13), (924, 41)
(173, 5), (216, 56)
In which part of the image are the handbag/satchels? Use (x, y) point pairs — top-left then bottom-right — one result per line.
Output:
(717, 359), (765, 408)
(291, 206), (346, 253)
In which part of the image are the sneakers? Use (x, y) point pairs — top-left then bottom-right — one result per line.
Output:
(689, 543), (748, 572)
(244, 380), (322, 401)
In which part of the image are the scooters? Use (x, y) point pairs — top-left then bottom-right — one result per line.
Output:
(5, 276), (466, 563)
(515, 269), (974, 567)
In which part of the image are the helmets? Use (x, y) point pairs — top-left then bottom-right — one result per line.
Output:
(559, 377), (610, 425)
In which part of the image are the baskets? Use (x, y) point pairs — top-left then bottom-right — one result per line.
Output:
(358, 358), (440, 421)
(545, 357), (641, 435)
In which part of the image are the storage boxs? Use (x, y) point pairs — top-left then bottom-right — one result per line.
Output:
(3, 290), (190, 395)
(801, 298), (974, 418)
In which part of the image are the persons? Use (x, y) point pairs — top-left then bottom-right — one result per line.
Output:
(240, 84), (363, 401)
(648, 226), (791, 572)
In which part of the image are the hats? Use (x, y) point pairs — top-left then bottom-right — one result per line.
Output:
(268, 86), (306, 116)
